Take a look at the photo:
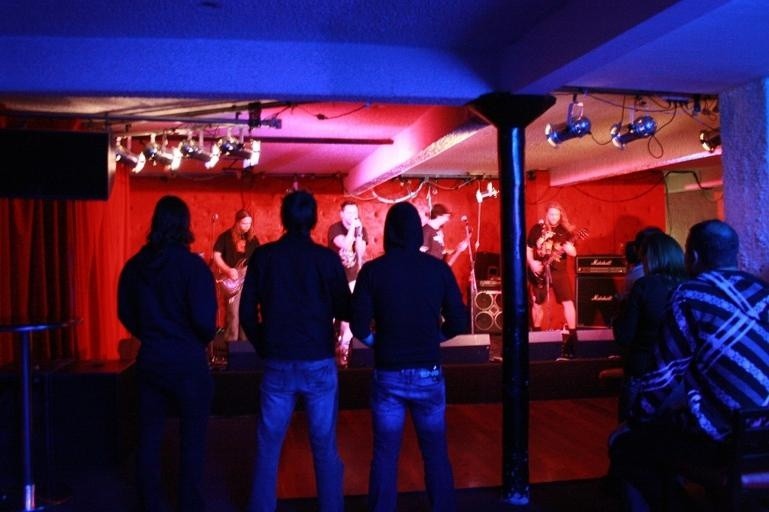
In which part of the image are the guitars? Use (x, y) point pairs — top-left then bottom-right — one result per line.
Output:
(445, 227), (473, 266)
(221, 252), (252, 297)
(527, 223), (591, 304)
(345, 234), (380, 284)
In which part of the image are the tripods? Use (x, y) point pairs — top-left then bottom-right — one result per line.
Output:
(53, 200), (107, 368)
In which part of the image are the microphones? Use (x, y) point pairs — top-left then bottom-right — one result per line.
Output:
(538, 218), (545, 237)
(461, 215), (469, 235)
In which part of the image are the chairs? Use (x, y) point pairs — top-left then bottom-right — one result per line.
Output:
(705, 406), (769, 512)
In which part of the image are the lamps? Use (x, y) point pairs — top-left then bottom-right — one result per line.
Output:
(547, 90), (658, 149)
(699, 129), (722, 153)
(114, 123), (260, 175)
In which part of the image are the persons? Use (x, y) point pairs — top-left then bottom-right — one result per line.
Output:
(234, 187), (354, 511)
(327, 199), (368, 295)
(417, 203), (472, 264)
(348, 201), (472, 511)
(611, 218), (769, 492)
(114, 194), (224, 512)
(520, 196), (582, 338)
(205, 207), (259, 339)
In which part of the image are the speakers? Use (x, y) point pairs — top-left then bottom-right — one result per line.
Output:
(440, 334), (491, 363)
(226, 342), (257, 370)
(564, 328), (616, 355)
(470, 291), (504, 335)
(348, 337), (375, 365)
(529, 331), (563, 361)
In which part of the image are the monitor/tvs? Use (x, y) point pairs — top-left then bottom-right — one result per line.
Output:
(0, 128), (109, 200)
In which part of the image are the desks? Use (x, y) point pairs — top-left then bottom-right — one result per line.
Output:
(0, 315), (78, 512)
